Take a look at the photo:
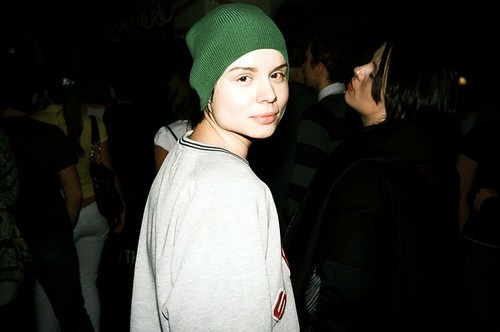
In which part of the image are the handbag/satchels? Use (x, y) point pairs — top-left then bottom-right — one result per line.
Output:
(89, 115), (124, 219)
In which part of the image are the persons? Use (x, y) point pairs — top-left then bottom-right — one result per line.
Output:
(102, 72), (151, 238)
(0, 53), (96, 332)
(129, 4), (302, 331)
(29, 52), (126, 332)
(440, 64), (482, 238)
(475, 187), (499, 224)
(281, 31), (454, 332)
(272, 37), (357, 284)
(153, 54), (207, 168)
(0, 121), (26, 331)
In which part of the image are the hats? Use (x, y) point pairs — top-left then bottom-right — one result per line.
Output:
(185, 3), (289, 110)
